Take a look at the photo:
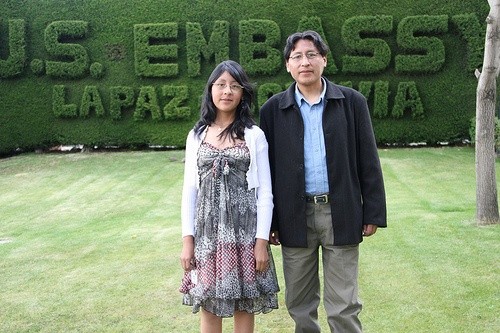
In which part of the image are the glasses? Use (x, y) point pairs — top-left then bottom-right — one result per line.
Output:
(211, 83), (244, 91)
(289, 52), (320, 61)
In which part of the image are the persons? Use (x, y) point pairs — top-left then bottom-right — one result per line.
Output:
(259, 30), (388, 333)
(179, 60), (281, 333)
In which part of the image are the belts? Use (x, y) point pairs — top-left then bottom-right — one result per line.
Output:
(307, 194), (329, 204)
